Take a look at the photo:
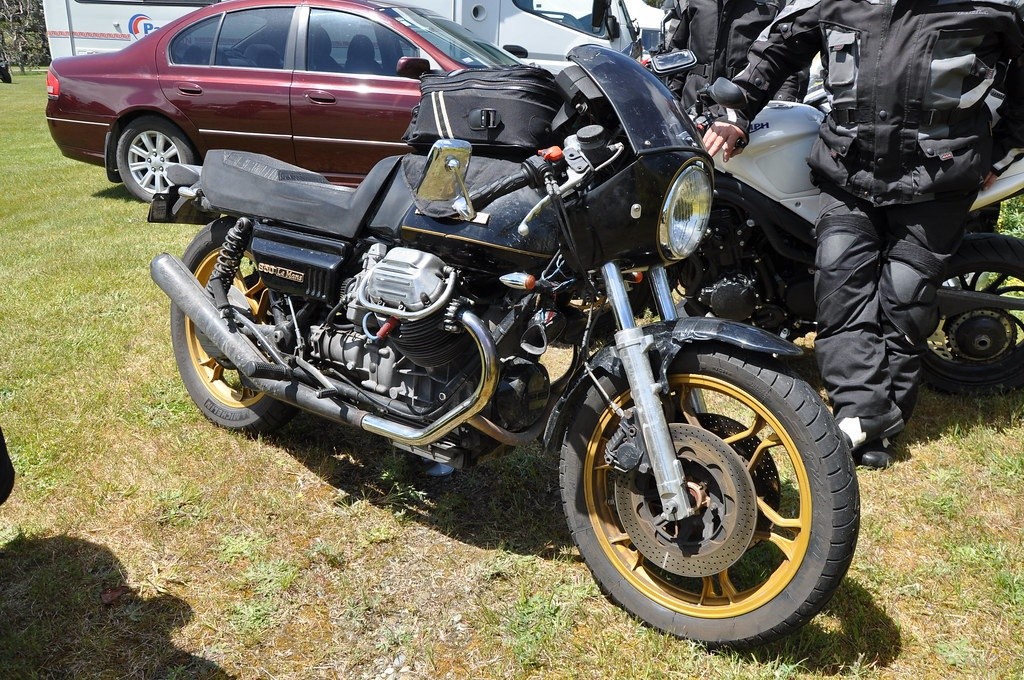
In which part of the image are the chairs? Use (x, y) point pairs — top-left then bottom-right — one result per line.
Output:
(345, 34), (383, 75)
(311, 26), (343, 71)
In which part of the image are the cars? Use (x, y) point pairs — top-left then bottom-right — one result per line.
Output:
(45, 2), (527, 204)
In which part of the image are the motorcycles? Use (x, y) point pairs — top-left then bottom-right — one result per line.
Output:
(147, 44), (1023, 650)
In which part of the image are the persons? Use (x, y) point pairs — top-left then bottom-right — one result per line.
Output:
(664, 0), (814, 120)
(702, 0), (1024, 472)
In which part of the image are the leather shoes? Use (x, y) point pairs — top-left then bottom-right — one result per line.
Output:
(836, 400), (905, 451)
(852, 437), (896, 468)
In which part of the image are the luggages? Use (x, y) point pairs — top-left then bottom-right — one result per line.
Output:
(400, 66), (564, 160)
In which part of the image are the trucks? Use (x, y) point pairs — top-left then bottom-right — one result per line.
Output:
(40, 1), (670, 76)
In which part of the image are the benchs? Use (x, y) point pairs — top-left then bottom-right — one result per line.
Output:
(184, 43), (281, 70)
